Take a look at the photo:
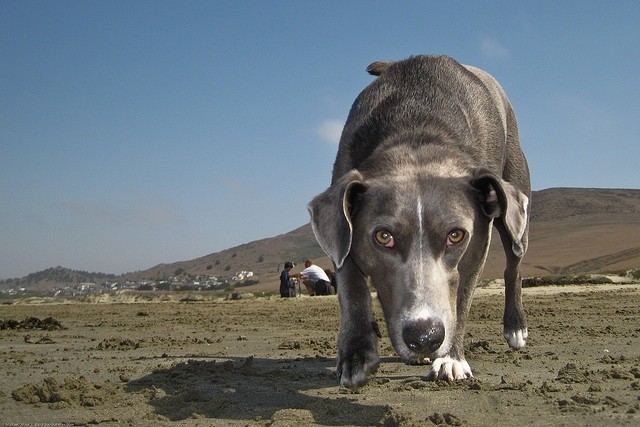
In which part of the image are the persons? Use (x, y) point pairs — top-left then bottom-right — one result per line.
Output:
(289, 259), (331, 296)
(279, 261), (296, 298)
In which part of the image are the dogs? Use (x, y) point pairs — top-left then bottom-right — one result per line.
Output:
(307, 54), (532, 390)
